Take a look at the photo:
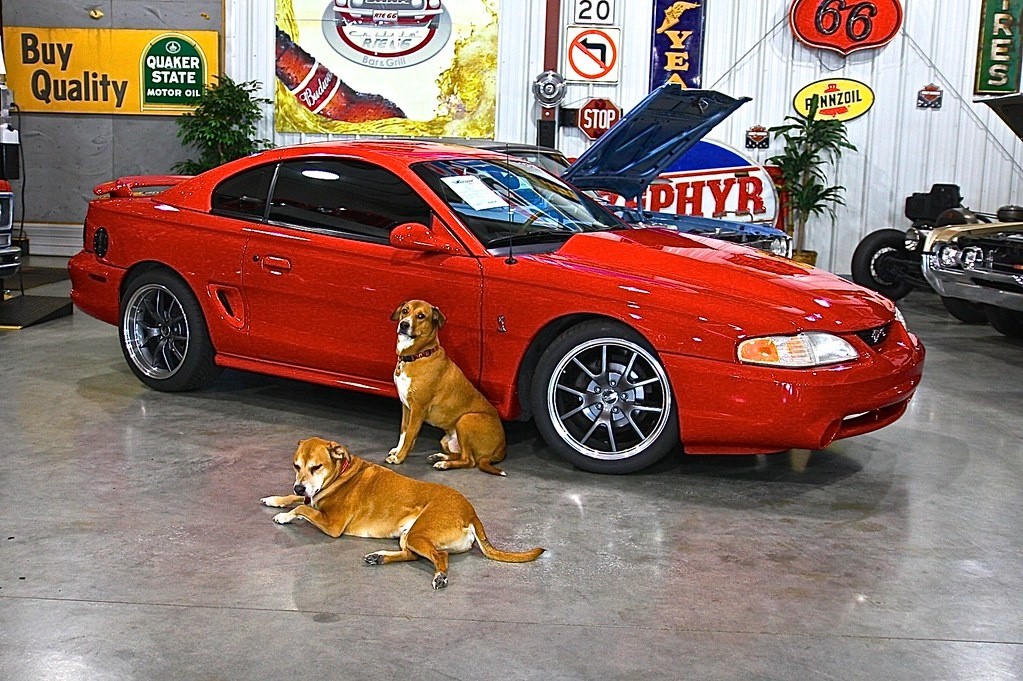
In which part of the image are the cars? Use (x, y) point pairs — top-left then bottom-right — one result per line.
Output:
(65, 140), (925, 480)
(439, 80), (791, 262)
(921, 219), (1022, 340)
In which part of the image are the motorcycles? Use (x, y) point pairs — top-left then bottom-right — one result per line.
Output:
(852, 182), (1014, 302)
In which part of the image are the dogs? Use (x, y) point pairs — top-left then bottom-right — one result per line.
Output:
(259, 438), (548, 590)
(381, 299), (508, 477)
